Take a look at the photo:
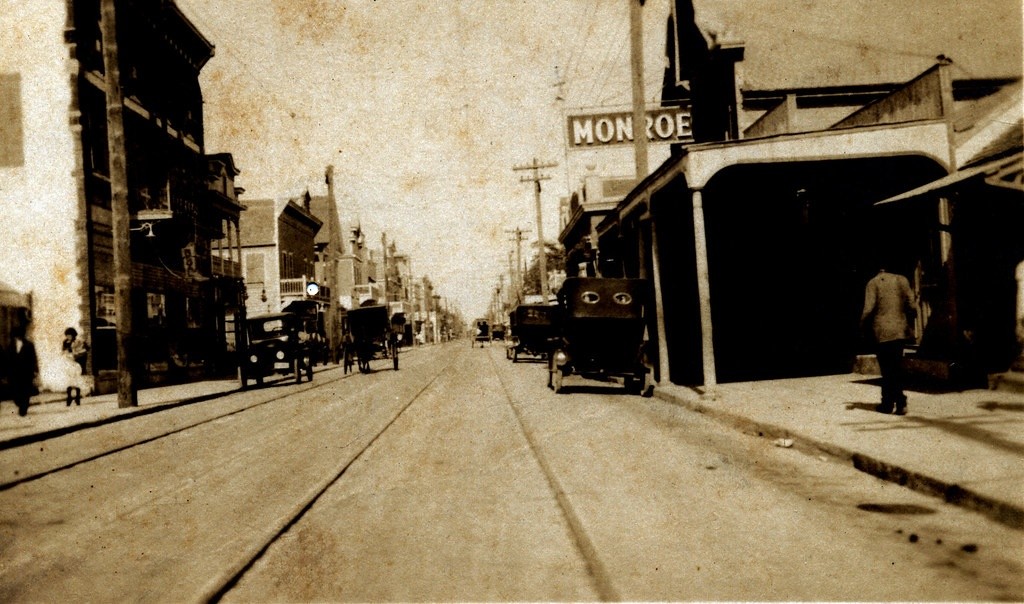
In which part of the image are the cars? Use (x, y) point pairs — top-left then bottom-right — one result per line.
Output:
(238, 310), (317, 387)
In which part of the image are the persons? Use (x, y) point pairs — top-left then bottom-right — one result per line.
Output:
(0, 328), (39, 417)
(62, 327), (90, 406)
(1015, 261), (1024, 340)
(478, 321), (489, 336)
(860, 267), (919, 414)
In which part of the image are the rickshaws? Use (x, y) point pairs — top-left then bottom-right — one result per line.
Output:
(348, 305), (401, 372)
(506, 301), (567, 365)
(545, 276), (658, 398)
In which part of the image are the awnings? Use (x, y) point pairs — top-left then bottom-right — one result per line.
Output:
(873, 152), (1021, 206)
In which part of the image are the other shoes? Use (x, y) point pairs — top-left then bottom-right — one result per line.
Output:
(876, 402), (893, 414)
(894, 406), (908, 416)
